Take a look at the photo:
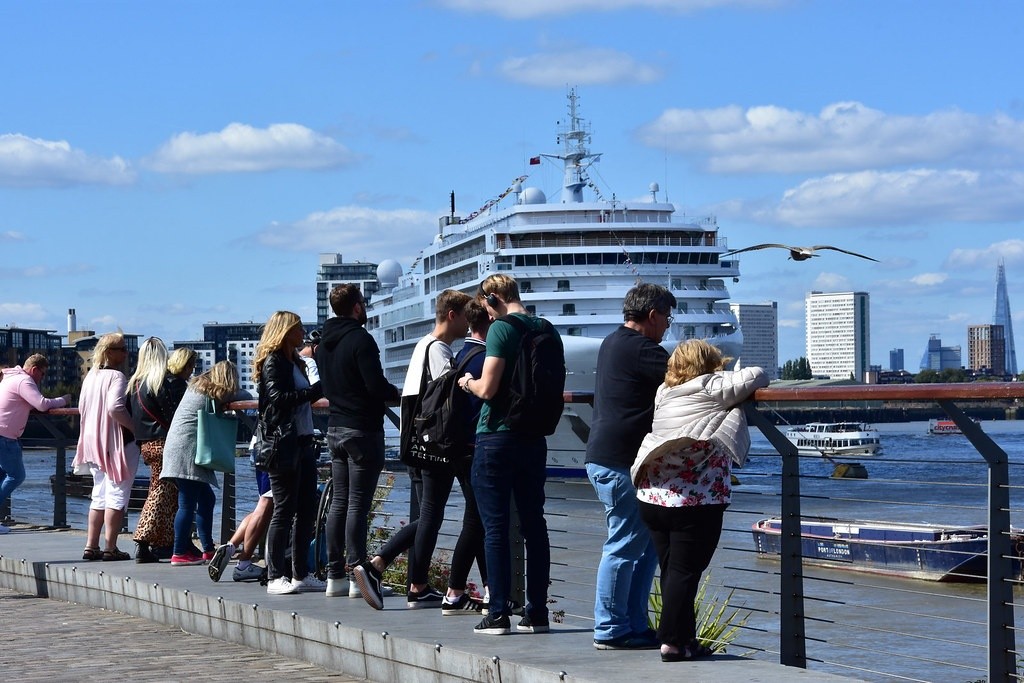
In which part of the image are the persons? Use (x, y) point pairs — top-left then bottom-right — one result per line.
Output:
(458, 272), (551, 635)
(249, 310), (326, 594)
(354, 290), (474, 612)
(315, 284), (402, 599)
(585, 284), (678, 652)
(0, 353), (72, 534)
(125, 337), (198, 563)
(71, 332), (140, 561)
(159, 361), (253, 565)
(441, 295), (523, 617)
(629, 338), (769, 663)
(208, 333), (329, 583)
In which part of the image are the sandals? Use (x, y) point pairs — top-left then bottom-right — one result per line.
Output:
(82, 546), (104, 560)
(660, 638), (711, 661)
(103, 546), (130, 561)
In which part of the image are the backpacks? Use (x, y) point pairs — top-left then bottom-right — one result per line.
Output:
(409, 345), (486, 464)
(489, 316), (566, 435)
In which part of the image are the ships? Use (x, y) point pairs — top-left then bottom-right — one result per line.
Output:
(363, 83), (741, 478)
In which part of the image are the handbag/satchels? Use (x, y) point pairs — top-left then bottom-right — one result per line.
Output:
(194, 397), (238, 472)
(399, 391), (424, 464)
(253, 417), (298, 470)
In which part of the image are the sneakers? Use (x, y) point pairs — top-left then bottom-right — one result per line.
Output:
(482, 596), (522, 615)
(291, 572), (327, 593)
(441, 593), (483, 615)
(267, 576), (297, 594)
(517, 617), (549, 632)
(353, 561), (384, 610)
(474, 614), (510, 634)
(408, 584), (443, 608)
(232, 563), (263, 581)
(208, 544), (232, 582)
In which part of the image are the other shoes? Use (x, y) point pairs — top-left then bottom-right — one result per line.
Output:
(326, 577), (348, 597)
(0, 524), (10, 534)
(152, 547), (173, 558)
(592, 627), (661, 650)
(202, 552), (215, 560)
(349, 578), (392, 598)
(134, 545), (158, 563)
(171, 554), (205, 565)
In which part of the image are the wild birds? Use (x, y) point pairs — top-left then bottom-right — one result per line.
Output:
(719, 243), (882, 262)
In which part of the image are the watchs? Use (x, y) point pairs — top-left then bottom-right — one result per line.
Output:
(462, 378), (475, 394)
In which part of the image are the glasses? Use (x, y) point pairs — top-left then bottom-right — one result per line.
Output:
(667, 313), (674, 325)
(362, 298), (369, 306)
(109, 345), (127, 352)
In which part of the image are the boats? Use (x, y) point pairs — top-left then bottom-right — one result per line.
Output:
(928, 411), (982, 434)
(751, 508), (1024, 582)
(49, 472), (149, 511)
(785, 422), (883, 455)
